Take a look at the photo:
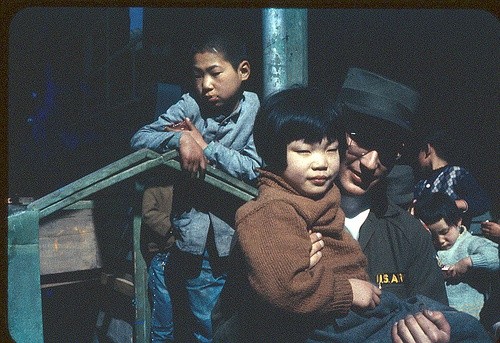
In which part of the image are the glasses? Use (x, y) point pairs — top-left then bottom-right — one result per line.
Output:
(335, 116), (413, 167)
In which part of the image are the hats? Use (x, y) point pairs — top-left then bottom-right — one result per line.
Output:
(332, 66), (420, 131)
